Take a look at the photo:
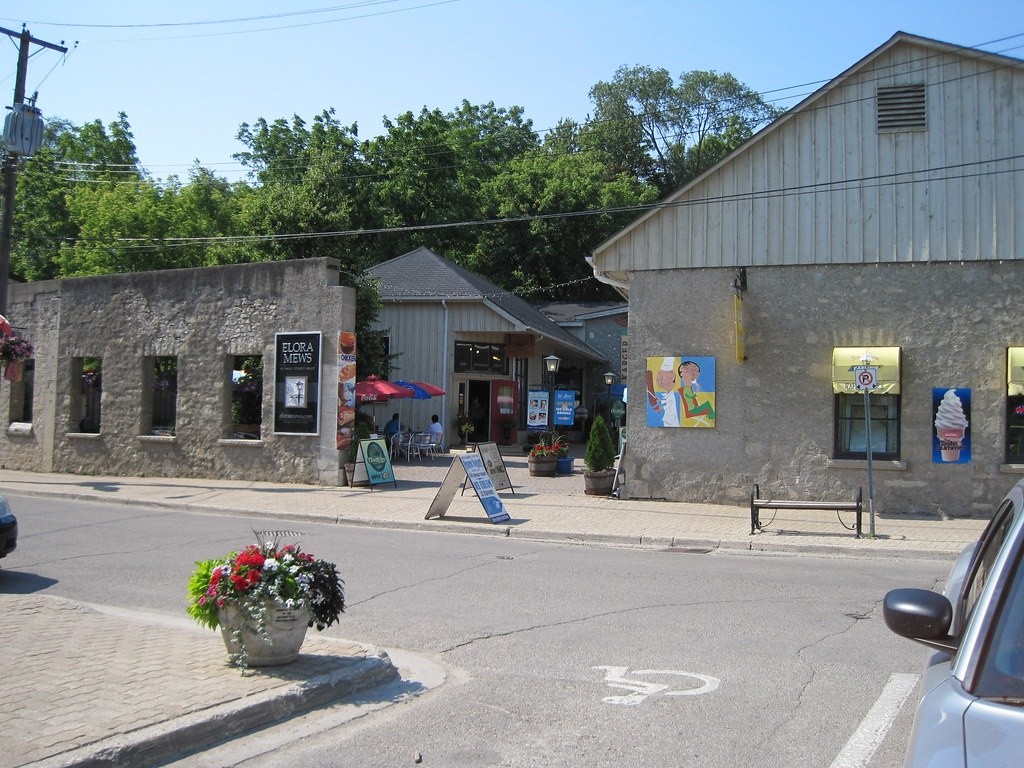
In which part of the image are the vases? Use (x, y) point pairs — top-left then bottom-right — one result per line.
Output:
(528, 457), (558, 477)
(216, 599), (309, 668)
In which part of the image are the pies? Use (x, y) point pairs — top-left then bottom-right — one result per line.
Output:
(338, 411), (355, 425)
(339, 363), (356, 382)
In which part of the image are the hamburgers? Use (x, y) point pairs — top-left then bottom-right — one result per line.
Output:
(338, 333), (355, 354)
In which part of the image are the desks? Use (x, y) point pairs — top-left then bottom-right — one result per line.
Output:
(400, 432), (423, 435)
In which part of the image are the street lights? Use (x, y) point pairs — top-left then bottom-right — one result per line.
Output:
(603, 371), (616, 440)
(543, 355), (562, 444)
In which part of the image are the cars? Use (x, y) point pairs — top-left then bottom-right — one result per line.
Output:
(879, 478), (1024, 768)
(0, 494), (18, 558)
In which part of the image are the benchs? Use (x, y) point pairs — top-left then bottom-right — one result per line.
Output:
(748, 484), (863, 539)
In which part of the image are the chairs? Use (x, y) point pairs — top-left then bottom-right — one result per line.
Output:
(385, 431), (445, 463)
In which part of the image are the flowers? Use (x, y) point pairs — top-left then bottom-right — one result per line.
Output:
(185, 535), (345, 671)
(530, 438), (561, 457)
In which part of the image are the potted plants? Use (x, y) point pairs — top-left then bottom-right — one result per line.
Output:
(559, 442), (573, 475)
(581, 414), (617, 495)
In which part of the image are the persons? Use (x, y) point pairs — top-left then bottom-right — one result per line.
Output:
(422, 415), (443, 457)
(384, 414), (402, 451)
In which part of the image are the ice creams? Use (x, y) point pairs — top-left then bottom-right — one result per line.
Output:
(935, 389), (969, 461)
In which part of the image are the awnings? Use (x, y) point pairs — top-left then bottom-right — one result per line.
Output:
(831, 346), (902, 396)
(1007, 347), (1024, 397)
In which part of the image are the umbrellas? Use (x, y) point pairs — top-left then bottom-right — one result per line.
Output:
(355, 374), (414, 433)
(393, 380), (432, 445)
(408, 381), (448, 431)
